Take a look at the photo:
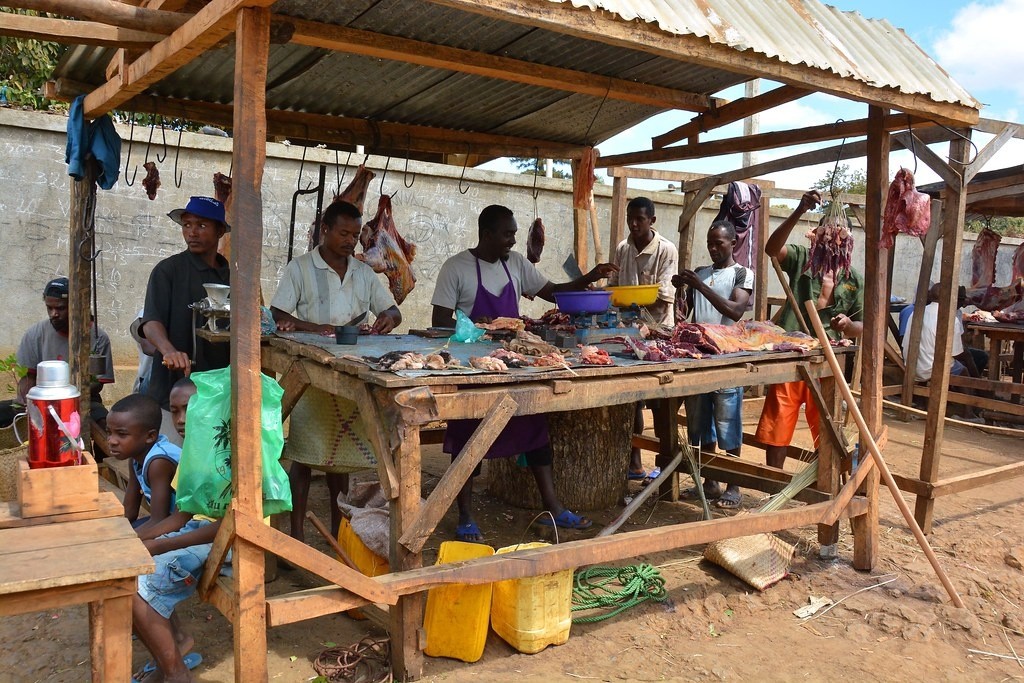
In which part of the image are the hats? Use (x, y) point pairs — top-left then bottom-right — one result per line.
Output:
(168, 197), (232, 233)
(42, 278), (68, 299)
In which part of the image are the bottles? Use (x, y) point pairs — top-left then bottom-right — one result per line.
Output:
(852, 443), (859, 475)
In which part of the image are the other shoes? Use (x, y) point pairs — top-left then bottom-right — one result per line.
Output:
(951, 415), (985, 424)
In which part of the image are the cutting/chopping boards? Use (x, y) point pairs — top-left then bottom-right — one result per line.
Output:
(409, 330), (516, 338)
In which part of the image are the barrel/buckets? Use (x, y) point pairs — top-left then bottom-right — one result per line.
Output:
(421, 541), (495, 662)
(491, 512), (573, 654)
(336, 517), (389, 619)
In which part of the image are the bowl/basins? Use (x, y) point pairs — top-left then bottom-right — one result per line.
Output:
(602, 284), (663, 305)
(553, 290), (613, 315)
(335, 326), (359, 345)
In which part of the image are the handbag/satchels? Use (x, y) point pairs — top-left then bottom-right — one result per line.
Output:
(175, 364), (294, 518)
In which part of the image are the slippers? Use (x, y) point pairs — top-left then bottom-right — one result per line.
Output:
(538, 509), (592, 528)
(132, 653), (202, 683)
(457, 522), (485, 543)
(716, 491), (743, 508)
(628, 467), (646, 479)
(679, 487), (724, 501)
(642, 468), (660, 486)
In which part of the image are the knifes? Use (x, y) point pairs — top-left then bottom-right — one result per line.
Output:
(343, 311), (367, 326)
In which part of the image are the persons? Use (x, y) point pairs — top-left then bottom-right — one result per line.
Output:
(899, 281), (934, 354)
(270, 201), (403, 572)
(132, 307), (155, 394)
(143, 195), (232, 409)
(604, 194), (679, 497)
(0, 278), (108, 466)
(671, 220), (754, 511)
(899, 282), (977, 419)
(106, 394), (181, 530)
(130, 376), (273, 683)
(754, 191), (864, 503)
(952, 285), (988, 379)
(431, 205), (621, 541)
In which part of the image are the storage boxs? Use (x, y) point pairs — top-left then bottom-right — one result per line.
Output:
(17, 454), (101, 519)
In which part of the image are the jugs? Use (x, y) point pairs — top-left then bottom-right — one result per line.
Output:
(13, 360), (85, 469)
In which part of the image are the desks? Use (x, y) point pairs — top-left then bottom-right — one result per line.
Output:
(765, 296), (909, 380)
(967, 323), (1024, 426)
(1, 496), (157, 683)
(194, 317), (860, 683)
